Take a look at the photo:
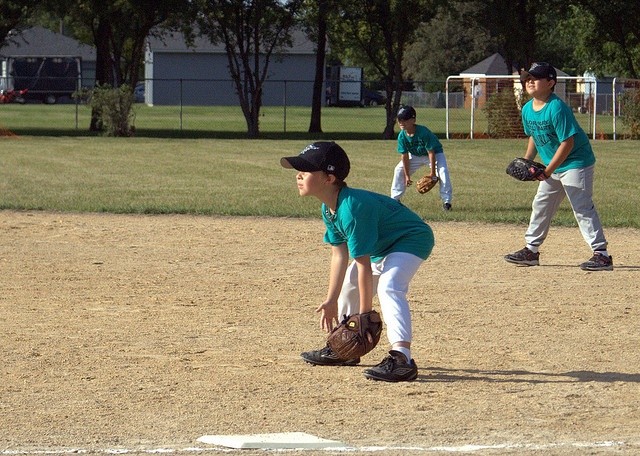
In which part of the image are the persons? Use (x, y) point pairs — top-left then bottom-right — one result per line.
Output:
(505, 64), (614, 270)
(280, 140), (434, 383)
(391, 105), (452, 211)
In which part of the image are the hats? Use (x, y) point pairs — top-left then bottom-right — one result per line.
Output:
(521, 64), (557, 80)
(280, 140), (350, 180)
(395, 104), (416, 118)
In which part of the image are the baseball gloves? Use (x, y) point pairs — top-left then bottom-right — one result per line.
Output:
(506, 157), (547, 181)
(415, 175), (440, 194)
(326, 310), (382, 362)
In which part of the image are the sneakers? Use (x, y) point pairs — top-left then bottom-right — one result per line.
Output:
(581, 252), (613, 270)
(300, 346), (360, 366)
(504, 248), (540, 265)
(364, 356), (419, 382)
(442, 202), (452, 210)
